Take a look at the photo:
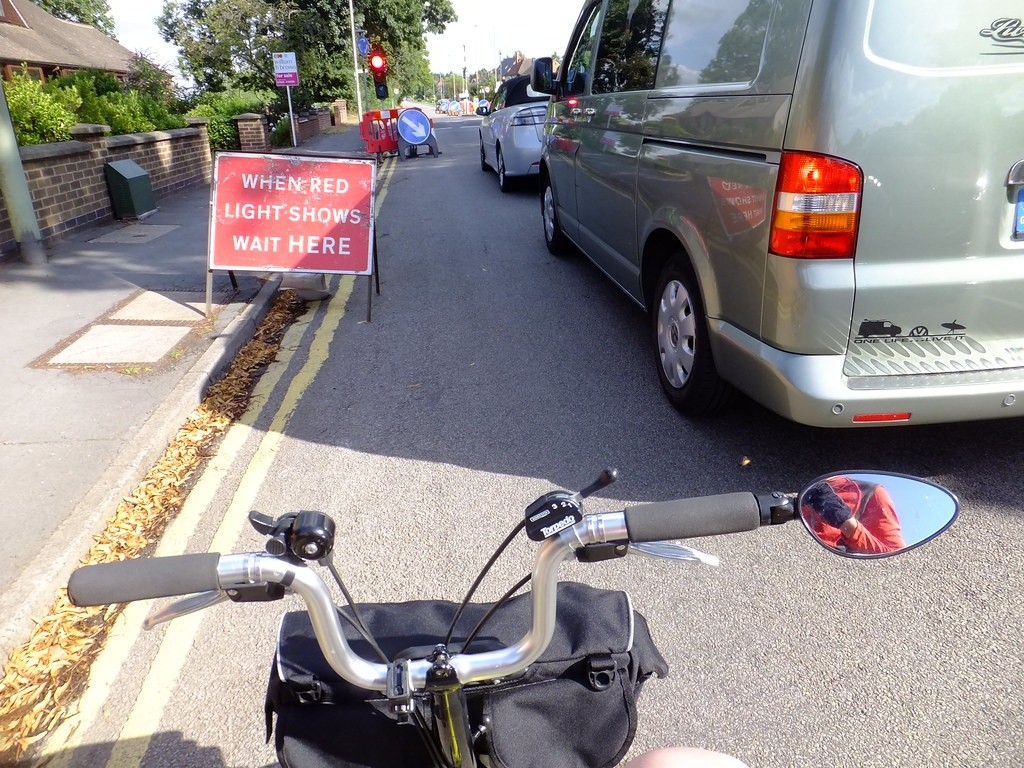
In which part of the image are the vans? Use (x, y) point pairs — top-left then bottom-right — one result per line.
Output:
(532, 0), (1024, 428)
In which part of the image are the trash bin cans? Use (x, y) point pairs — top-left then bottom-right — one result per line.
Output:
(104, 158), (159, 222)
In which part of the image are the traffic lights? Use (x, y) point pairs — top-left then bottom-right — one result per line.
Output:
(371, 54), (389, 99)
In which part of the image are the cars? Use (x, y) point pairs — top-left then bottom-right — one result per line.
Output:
(476, 72), (561, 193)
